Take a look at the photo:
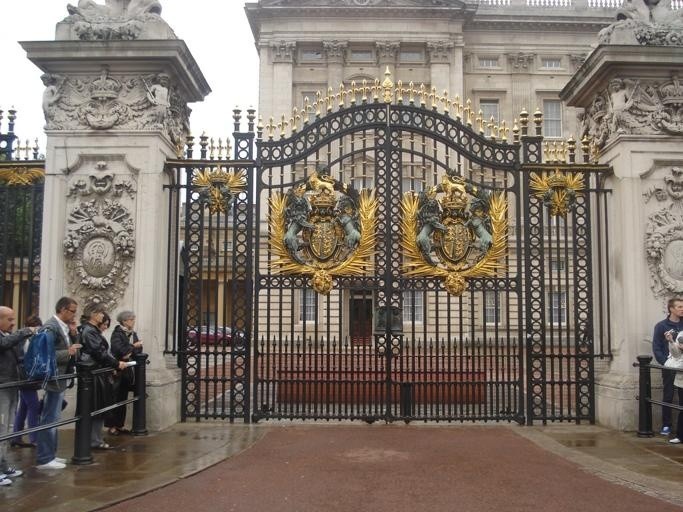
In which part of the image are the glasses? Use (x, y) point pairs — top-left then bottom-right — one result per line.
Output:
(65, 307), (76, 313)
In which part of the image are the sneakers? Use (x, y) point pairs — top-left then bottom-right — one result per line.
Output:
(0, 467), (22, 485)
(108, 427), (128, 436)
(660, 426), (672, 435)
(669, 438), (681, 443)
(35, 457), (66, 469)
(91, 441), (113, 449)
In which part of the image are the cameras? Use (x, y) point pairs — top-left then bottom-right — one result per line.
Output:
(668, 329), (677, 339)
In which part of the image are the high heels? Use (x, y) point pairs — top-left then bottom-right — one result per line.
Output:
(11, 441), (32, 447)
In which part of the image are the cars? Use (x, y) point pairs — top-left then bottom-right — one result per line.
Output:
(217, 326), (246, 345)
(186, 325), (237, 346)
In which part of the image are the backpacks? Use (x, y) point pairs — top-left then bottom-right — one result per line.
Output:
(23, 326), (59, 389)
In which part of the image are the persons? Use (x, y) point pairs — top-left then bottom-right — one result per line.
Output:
(147, 73), (172, 128)
(39, 73), (59, 126)
(652, 298), (683, 436)
(103, 310), (144, 436)
(663, 329), (683, 444)
(607, 77), (640, 134)
(0, 296), (128, 485)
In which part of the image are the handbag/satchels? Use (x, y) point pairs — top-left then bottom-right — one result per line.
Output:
(664, 352), (683, 369)
